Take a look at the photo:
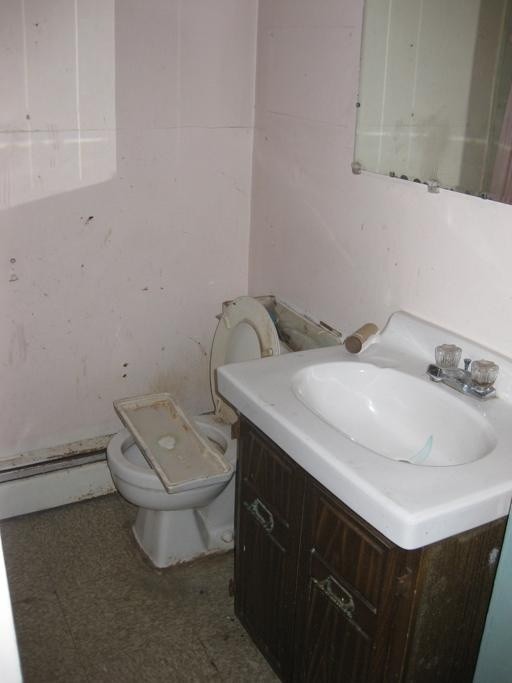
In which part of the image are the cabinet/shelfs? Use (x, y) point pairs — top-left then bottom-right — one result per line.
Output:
(233, 413), (509, 681)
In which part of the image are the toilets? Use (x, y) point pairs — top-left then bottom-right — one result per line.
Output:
(107, 297), (281, 568)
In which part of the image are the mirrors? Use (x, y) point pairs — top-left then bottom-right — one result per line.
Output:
(351, 0), (512, 204)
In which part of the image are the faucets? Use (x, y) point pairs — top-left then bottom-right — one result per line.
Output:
(424, 344), (500, 401)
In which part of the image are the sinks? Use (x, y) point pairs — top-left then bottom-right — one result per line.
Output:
(216, 310), (511, 522)
